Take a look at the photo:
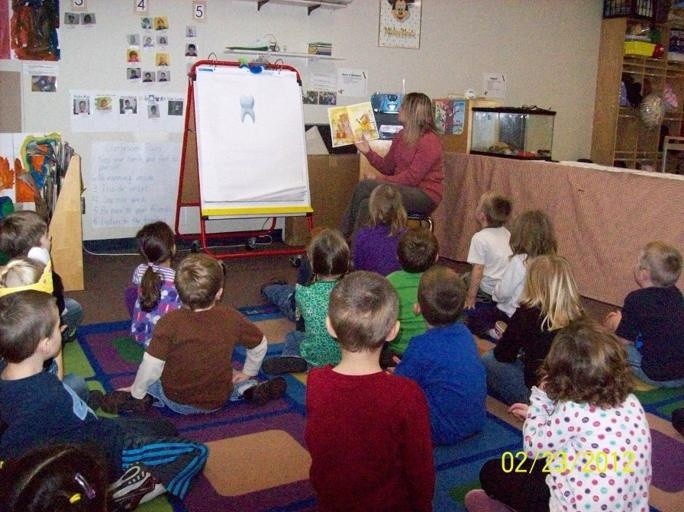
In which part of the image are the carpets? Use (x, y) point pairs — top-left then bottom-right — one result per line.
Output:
(46, 305), (684, 512)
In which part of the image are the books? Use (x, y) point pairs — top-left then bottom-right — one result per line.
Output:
(328, 102), (379, 148)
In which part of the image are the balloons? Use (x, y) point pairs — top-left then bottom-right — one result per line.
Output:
(638, 93), (664, 130)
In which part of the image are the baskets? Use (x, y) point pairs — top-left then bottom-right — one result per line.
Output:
(624, 41), (656, 56)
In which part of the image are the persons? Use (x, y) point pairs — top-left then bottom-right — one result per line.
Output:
(462, 208), (558, 342)
(482, 256), (592, 409)
(0, 290), (209, 509)
(9, 1), (197, 124)
(391, 265), (488, 447)
(340, 93), (445, 239)
(464, 320), (651, 511)
(262, 246), (313, 322)
(609, 241), (684, 388)
(465, 191), (519, 308)
(259, 230), (349, 376)
(385, 227), (440, 366)
(307, 271), (435, 511)
(110, 251), (286, 416)
(350, 183), (408, 276)
(0, 211), (89, 402)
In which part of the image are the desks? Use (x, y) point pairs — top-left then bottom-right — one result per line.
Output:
(433, 151), (684, 311)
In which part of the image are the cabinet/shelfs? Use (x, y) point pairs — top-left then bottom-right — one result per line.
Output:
(217, 0), (346, 67)
(588, 15), (683, 174)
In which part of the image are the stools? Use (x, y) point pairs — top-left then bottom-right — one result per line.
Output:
(407, 212), (433, 233)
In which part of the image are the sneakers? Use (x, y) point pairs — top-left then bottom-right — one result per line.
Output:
(463, 489), (518, 512)
(88, 390), (153, 414)
(106, 462), (160, 512)
(495, 320), (508, 338)
(243, 356), (307, 405)
(261, 280), (287, 294)
